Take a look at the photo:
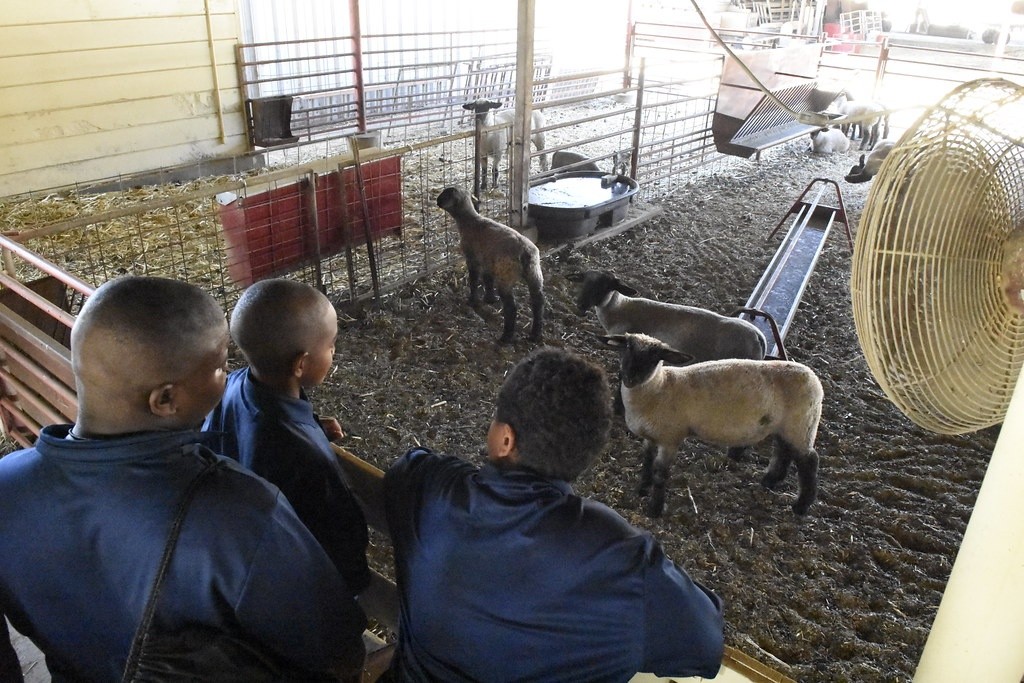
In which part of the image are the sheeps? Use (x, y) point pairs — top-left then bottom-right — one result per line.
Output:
(593, 333), (825, 519)
(803, 87), (895, 184)
(565, 270), (767, 416)
(436, 186), (546, 345)
(462, 101), (549, 190)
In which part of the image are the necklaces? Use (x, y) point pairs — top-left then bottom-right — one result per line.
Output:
(69, 428), (96, 441)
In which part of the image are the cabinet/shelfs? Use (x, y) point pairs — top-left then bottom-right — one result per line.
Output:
(218, 155), (403, 290)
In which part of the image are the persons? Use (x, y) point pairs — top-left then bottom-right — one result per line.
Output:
(197, 277), (369, 595)
(378, 347), (725, 682)
(1, 275), (370, 683)
